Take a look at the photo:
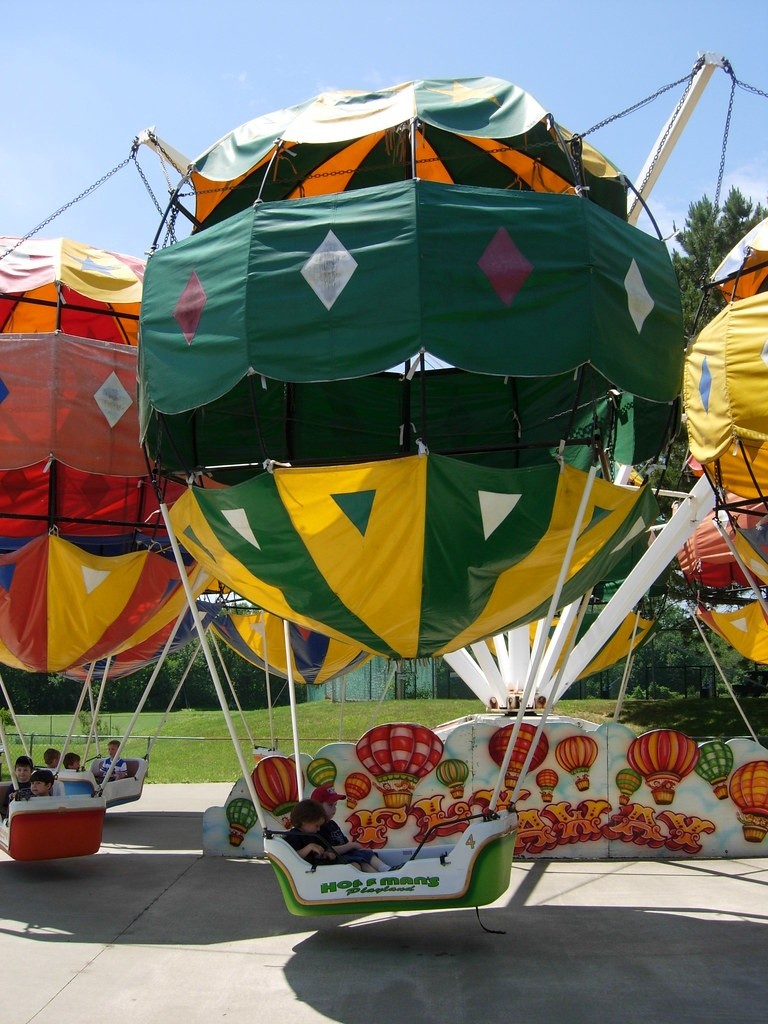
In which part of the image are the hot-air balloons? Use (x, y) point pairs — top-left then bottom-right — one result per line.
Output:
(684, 212), (767, 743)
(0, 236), (231, 862)
(136, 73), (687, 916)
(209, 611), (376, 761)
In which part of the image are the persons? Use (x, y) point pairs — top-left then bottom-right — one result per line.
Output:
(283, 798), (361, 872)
(94, 740), (128, 785)
(311, 784), (405, 873)
(0, 748), (81, 827)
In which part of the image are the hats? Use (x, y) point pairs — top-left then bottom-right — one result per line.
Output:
(311, 786), (346, 804)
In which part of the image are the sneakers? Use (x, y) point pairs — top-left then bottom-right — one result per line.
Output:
(386, 862), (406, 871)
